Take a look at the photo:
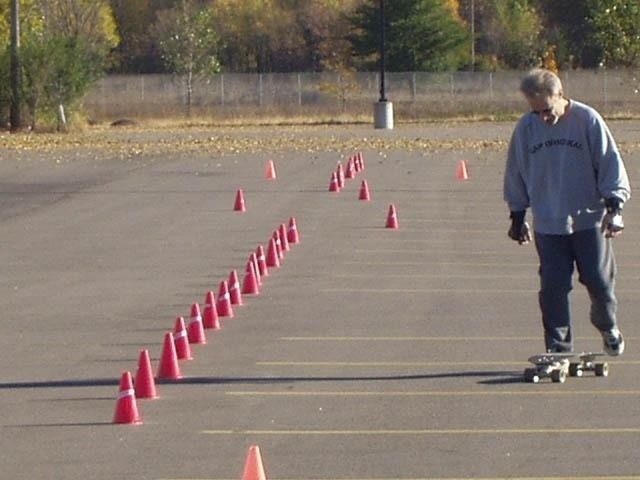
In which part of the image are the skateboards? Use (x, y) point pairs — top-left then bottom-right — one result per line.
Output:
(522, 351), (609, 383)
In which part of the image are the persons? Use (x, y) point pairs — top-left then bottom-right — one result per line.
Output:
(499, 65), (633, 359)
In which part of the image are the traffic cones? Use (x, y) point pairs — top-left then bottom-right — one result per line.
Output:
(265, 160), (276, 178)
(386, 204), (398, 228)
(234, 189), (245, 211)
(242, 445), (266, 479)
(359, 179), (369, 199)
(112, 217), (300, 424)
(330, 152), (365, 193)
(458, 161), (467, 179)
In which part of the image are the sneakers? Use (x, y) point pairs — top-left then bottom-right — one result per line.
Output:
(601, 327), (624, 356)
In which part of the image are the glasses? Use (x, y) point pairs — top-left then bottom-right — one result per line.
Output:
(530, 106), (554, 115)
